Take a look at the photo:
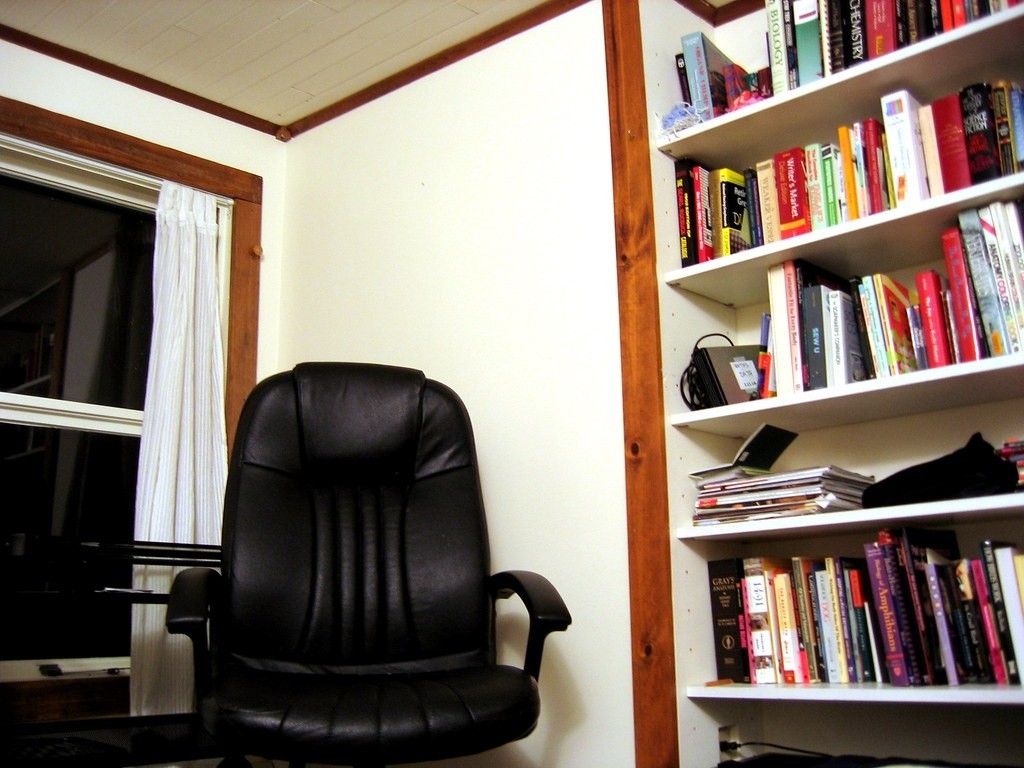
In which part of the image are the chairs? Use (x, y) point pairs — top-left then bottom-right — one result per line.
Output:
(164, 362), (573, 768)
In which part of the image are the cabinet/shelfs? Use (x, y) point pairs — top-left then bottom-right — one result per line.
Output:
(602, 0), (1024, 768)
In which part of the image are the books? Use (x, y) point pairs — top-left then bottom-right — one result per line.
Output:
(674, 0), (1024, 691)
